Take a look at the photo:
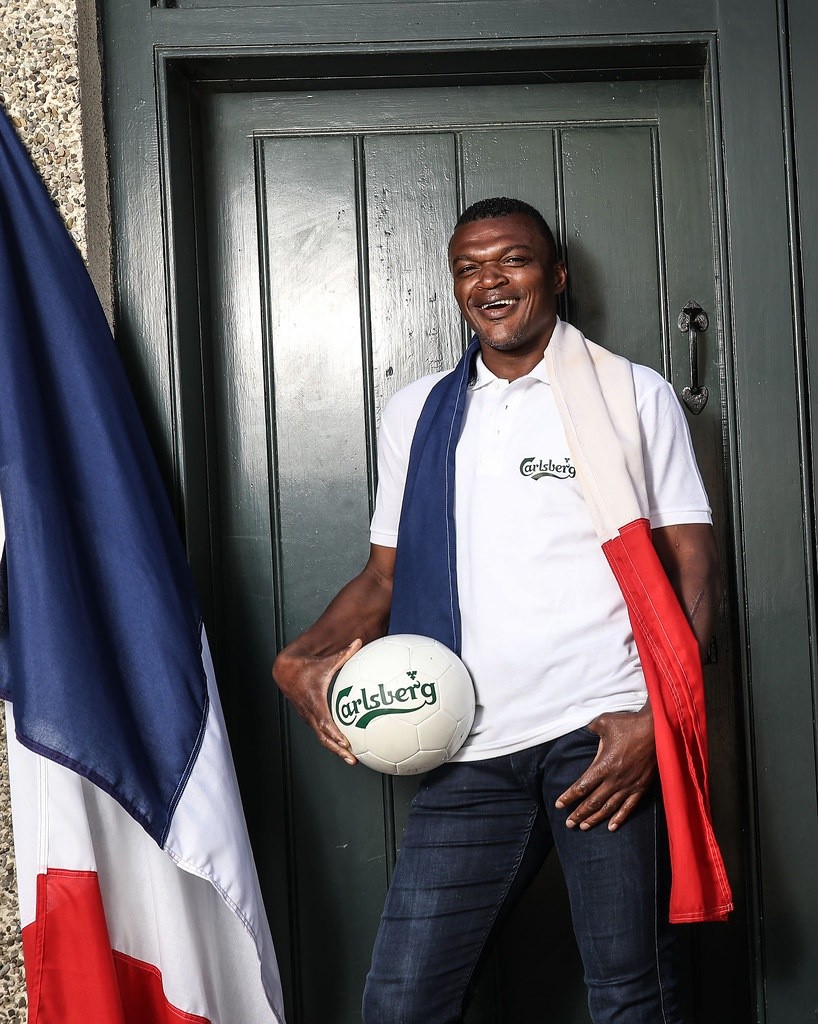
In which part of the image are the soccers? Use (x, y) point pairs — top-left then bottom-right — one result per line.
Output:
(330, 633), (477, 776)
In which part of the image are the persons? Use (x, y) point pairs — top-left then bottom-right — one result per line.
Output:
(271, 196), (724, 1024)
